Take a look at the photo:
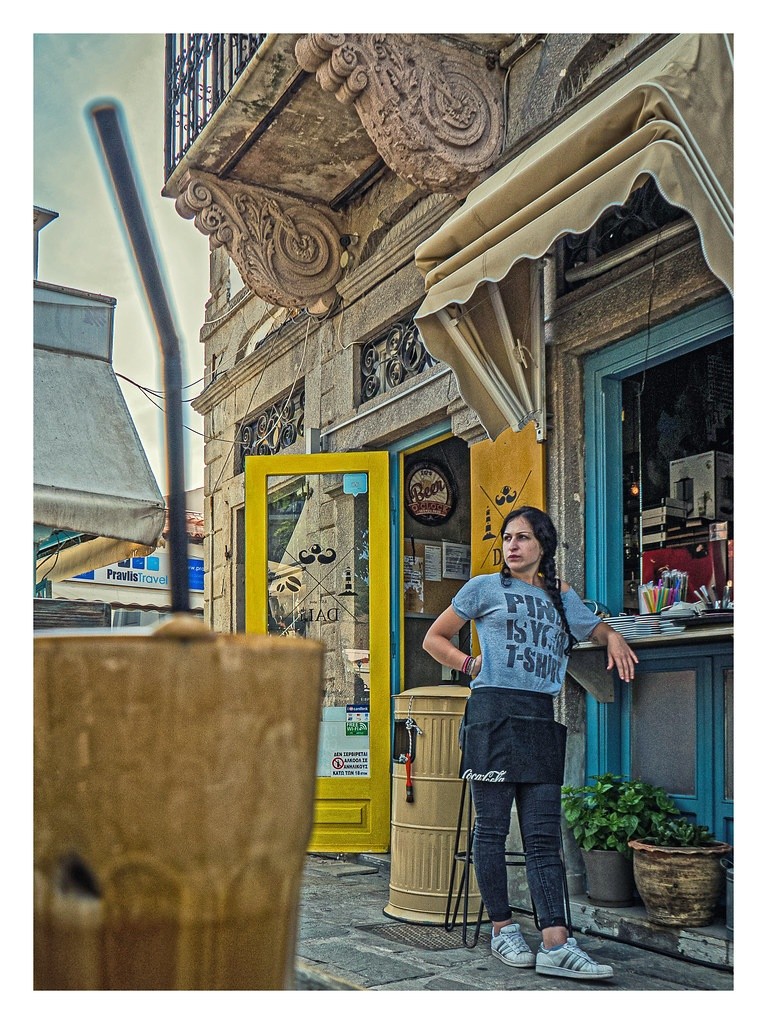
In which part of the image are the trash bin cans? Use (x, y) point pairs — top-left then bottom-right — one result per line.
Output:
(381, 683), (493, 926)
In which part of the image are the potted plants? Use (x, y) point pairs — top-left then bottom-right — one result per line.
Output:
(628, 788), (732, 926)
(561, 770), (682, 908)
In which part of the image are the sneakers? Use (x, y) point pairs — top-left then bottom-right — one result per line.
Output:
(535, 936), (615, 980)
(490, 923), (537, 968)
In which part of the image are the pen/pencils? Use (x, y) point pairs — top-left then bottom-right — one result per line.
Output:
(721, 585), (730, 608)
(694, 590), (710, 610)
(710, 584), (721, 609)
(700, 584), (713, 609)
(638, 569), (689, 614)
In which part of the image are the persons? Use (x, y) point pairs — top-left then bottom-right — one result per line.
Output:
(421, 505), (639, 978)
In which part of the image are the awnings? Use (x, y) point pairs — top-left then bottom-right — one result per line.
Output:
(414, 35), (731, 446)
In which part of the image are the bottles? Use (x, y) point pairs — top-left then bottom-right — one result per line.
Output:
(623, 514), (631, 556)
(631, 516), (639, 555)
